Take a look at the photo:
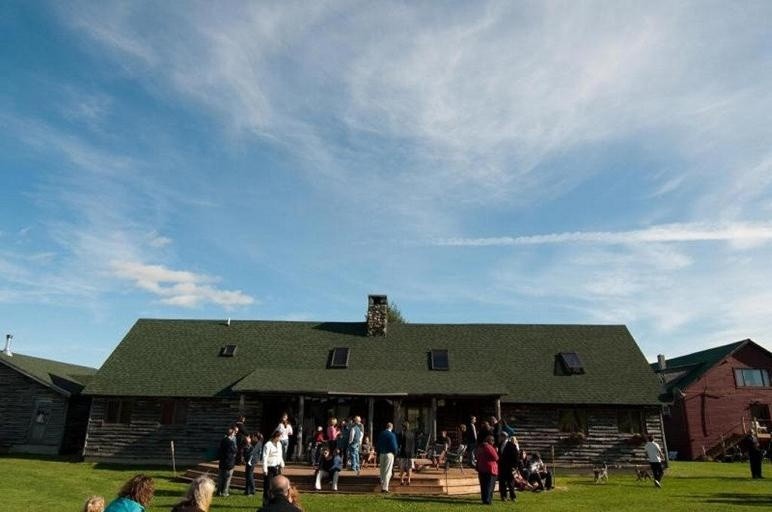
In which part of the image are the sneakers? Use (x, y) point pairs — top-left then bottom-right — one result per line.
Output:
(653, 478), (661, 489)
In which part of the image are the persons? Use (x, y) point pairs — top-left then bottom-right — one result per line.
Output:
(171, 474), (215, 511)
(646, 435), (666, 488)
(256, 474), (305, 512)
(399, 421), (416, 486)
(218, 428), (238, 496)
(430, 430), (451, 460)
(496, 431), (521, 502)
(474, 434), (499, 506)
(84, 495), (105, 512)
(229, 410), (375, 491)
(743, 427), (765, 479)
(108, 473), (157, 512)
(376, 422), (399, 493)
(453, 417), (554, 491)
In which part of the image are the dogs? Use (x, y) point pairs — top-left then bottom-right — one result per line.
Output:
(593, 461), (608, 483)
(632, 465), (653, 482)
(607, 465), (624, 474)
